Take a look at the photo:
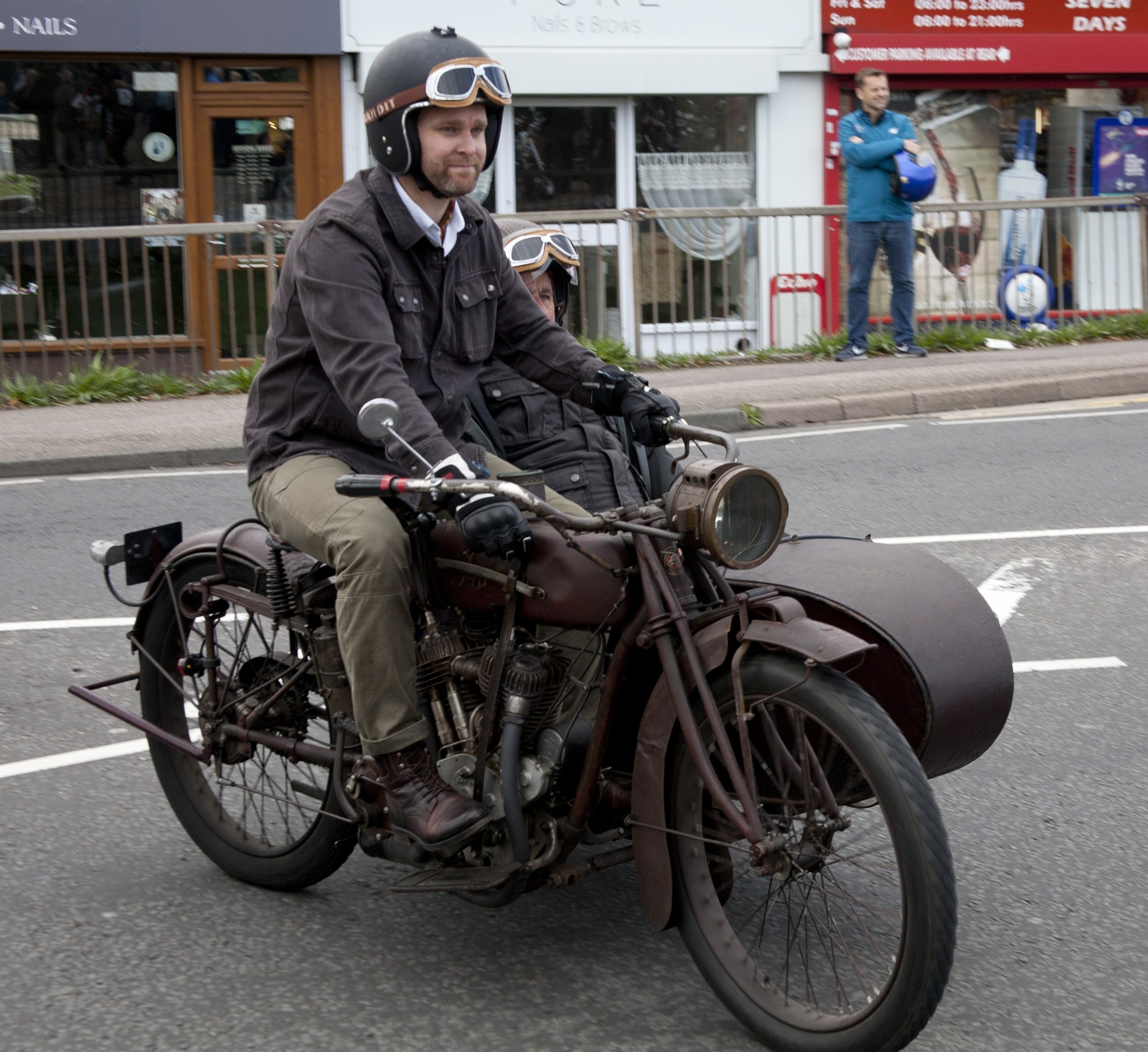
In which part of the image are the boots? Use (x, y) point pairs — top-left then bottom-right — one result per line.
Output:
(374, 739), (493, 851)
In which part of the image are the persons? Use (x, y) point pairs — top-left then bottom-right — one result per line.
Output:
(458, 215), (690, 518)
(242, 25), (684, 850)
(837, 69), (928, 359)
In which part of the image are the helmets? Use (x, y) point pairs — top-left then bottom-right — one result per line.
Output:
(894, 151), (937, 202)
(493, 218), (571, 327)
(364, 25), (504, 177)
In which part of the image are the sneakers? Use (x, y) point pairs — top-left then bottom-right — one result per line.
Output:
(894, 340), (926, 358)
(834, 342), (868, 362)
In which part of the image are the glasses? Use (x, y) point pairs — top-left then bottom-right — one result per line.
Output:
(501, 230), (580, 273)
(425, 57), (513, 108)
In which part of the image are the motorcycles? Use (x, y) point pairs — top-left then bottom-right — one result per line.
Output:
(68, 396), (1015, 1052)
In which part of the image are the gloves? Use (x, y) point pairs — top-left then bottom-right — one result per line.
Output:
(422, 453), (534, 562)
(586, 362), (680, 448)
